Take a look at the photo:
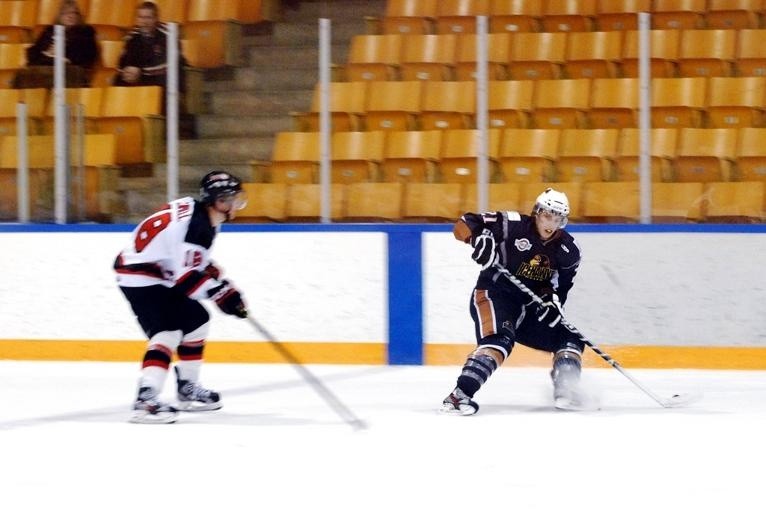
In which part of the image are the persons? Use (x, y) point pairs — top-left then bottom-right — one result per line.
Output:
(112, 169), (250, 415)
(109, 0), (185, 86)
(13, 0), (101, 87)
(441, 186), (585, 412)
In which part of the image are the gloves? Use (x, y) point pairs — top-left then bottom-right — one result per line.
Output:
(207, 279), (250, 319)
(537, 294), (562, 328)
(472, 228), (497, 269)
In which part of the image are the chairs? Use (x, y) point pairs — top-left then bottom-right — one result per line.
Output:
(1, 0), (766, 224)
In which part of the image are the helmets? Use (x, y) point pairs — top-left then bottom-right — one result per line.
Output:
(531, 187), (570, 216)
(199, 171), (242, 204)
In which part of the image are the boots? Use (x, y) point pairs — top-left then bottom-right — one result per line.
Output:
(553, 382), (581, 406)
(174, 365), (220, 403)
(443, 386), (479, 414)
(132, 387), (176, 412)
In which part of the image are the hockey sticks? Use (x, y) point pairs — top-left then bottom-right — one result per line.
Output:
(464, 233), (690, 411)
(235, 307), (369, 433)
